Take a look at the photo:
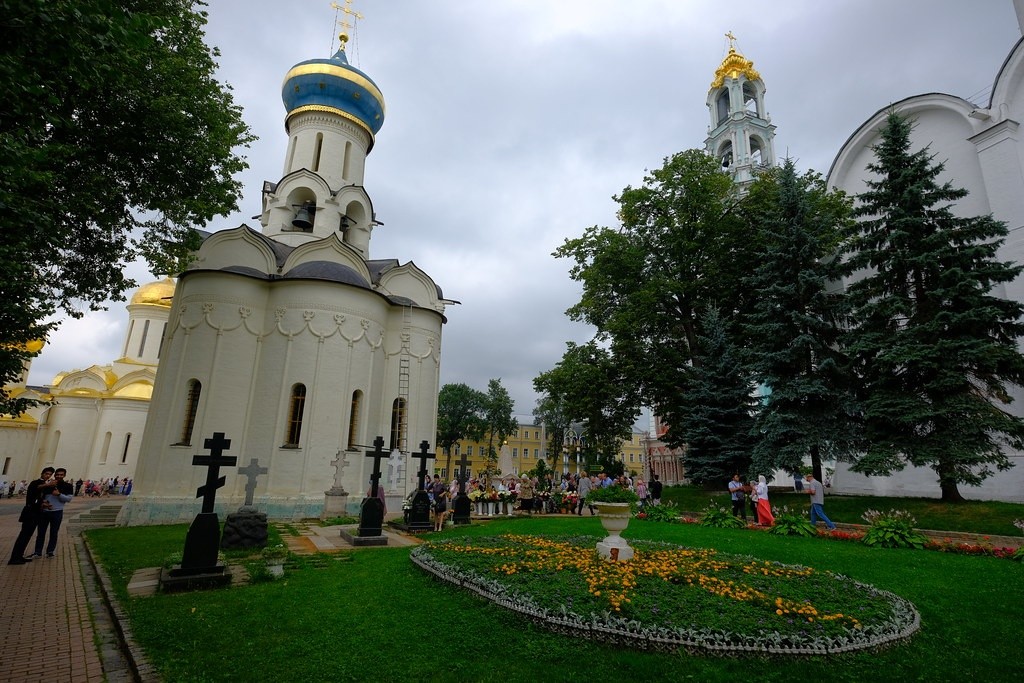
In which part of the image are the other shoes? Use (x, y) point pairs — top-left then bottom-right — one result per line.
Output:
(8, 558), (32, 565)
(646, 495), (650, 499)
(753, 522), (757, 524)
(756, 523), (763, 525)
(591, 513), (595, 515)
(578, 513), (582, 516)
(832, 528), (837, 532)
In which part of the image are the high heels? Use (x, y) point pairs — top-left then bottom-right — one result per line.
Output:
(434, 528), (442, 531)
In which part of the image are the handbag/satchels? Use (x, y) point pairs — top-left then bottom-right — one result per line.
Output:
(736, 489), (745, 502)
(19, 506), (28, 522)
(436, 497), (446, 513)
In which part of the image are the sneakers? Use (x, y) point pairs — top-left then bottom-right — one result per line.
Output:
(25, 553), (42, 559)
(47, 552), (55, 558)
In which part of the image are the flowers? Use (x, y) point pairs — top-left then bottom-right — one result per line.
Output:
(467, 490), (518, 504)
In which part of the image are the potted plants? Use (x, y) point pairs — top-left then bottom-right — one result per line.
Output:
(584, 482), (642, 561)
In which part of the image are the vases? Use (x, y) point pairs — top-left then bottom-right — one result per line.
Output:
(493, 503), (496, 514)
(477, 502), (483, 515)
(498, 502), (503, 515)
(507, 503), (513, 515)
(488, 503), (493, 516)
(484, 503), (488, 514)
(474, 502), (477, 513)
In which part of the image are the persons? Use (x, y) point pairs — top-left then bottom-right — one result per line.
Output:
(803, 474), (837, 532)
(825, 477), (831, 488)
(752, 474), (776, 526)
(0, 480), (29, 499)
(46, 475), (133, 498)
(728, 474), (747, 520)
(794, 475), (803, 492)
(367, 478), (387, 518)
(8, 467), (58, 565)
(26, 468), (73, 560)
(419, 468), (662, 532)
(749, 480), (760, 525)
